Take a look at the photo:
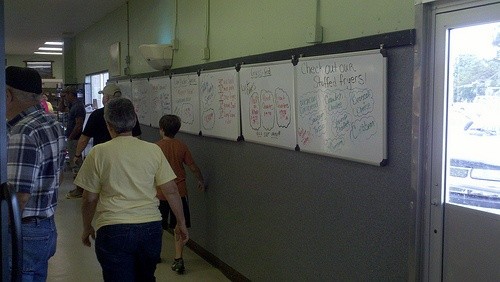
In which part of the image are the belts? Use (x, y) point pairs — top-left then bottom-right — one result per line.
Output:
(21, 216), (45, 222)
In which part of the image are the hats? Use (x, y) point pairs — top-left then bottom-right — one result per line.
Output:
(61, 86), (77, 94)
(98, 84), (121, 97)
(5, 66), (43, 94)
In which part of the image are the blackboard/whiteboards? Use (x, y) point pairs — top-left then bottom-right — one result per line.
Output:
(197, 67), (239, 141)
(238, 59), (295, 152)
(295, 50), (389, 167)
(105, 72), (198, 136)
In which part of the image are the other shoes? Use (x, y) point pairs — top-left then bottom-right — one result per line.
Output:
(69, 190), (82, 197)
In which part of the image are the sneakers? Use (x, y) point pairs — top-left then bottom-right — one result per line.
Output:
(170, 259), (185, 273)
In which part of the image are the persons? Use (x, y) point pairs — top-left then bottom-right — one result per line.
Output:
(62, 86), (86, 199)
(72, 81), (142, 167)
(73, 97), (190, 282)
(4, 66), (68, 282)
(153, 114), (205, 274)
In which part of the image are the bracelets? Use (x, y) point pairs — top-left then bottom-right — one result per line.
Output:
(74, 155), (81, 158)
